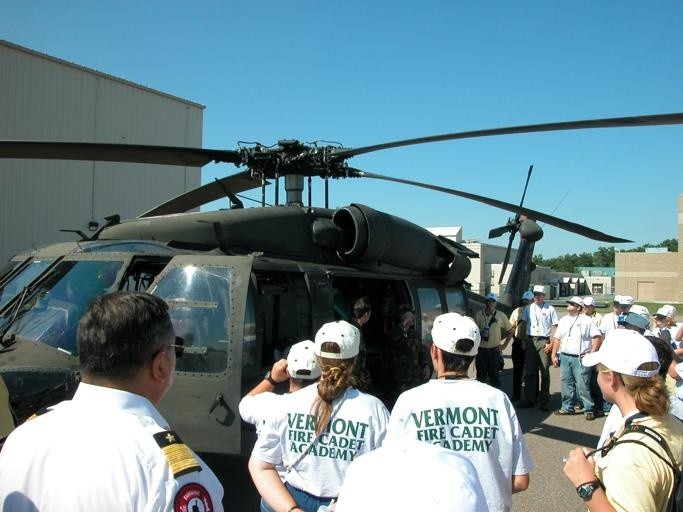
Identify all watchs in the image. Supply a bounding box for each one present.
[577,481,600,499]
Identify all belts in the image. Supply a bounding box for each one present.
[562,353,579,357]
[529,336,549,340]
[479,347,497,351]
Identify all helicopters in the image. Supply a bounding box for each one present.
[0,112,683,512]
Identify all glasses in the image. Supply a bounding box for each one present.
[166,336,184,358]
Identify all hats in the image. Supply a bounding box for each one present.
[533,285,545,295]
[485,293,497,302]
[315,320,360,359]
[583,297,595,307]
[427,312,481,356]
[566,296,584,309]
[620,296,634,305]
[613,295,622,304]
[286,340,322,380]
[522,291,534,300]
[624,305,650,324]
[582,329,661,377]
[617,312,650,330]
[663,305,676,316]
[653,308,670,318]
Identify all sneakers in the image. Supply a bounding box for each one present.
[595,409,603,418]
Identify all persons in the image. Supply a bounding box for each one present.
[552,295,603,420]
[248,321,391,511]
[509,291,535,402]
[476,293,514,386]
[383,311,531,511]
[616,312,648,337]
[599,294,622,341]
[520,284,558,410]
[563,329,682,511]
[238,338,321,483]
[352,298,371,388]
[2,290,224,511]
[394,307,433,396]
[584,297,605,417]
[651,307,674,341]
[617,296,633,315]
[595,335,682,463]
[628,305,649,322]
[661,305,682,330]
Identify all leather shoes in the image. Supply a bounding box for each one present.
[585,412,594,419]
[554,409,575,415]
[517,401,535,409]
[510,396,520,402]
[535,396,539,402]
[540,403,549,412]
